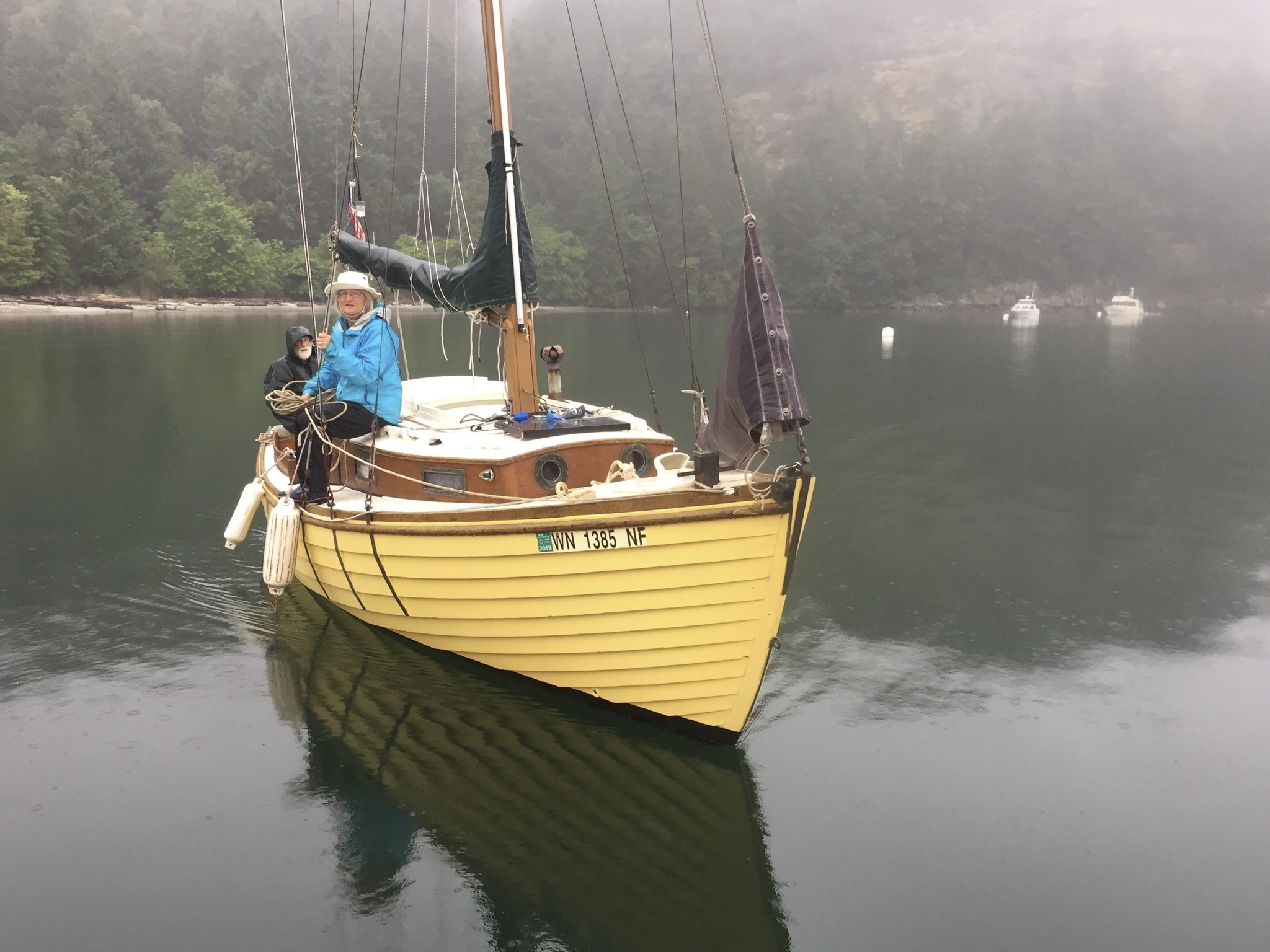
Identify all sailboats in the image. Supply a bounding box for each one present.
[256,2,825,750]
[260,566,793,952]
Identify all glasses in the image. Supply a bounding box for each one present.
[336,290,364,299]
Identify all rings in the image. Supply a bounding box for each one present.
[319,341,322,345]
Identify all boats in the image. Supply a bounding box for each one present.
[1105,287,1145,317]
[1011,295,1040,313]
[882,326,894,337]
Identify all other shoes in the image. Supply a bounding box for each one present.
[278,487,329,504]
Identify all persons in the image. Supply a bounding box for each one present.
[261,326,321,434]
[286,271,403,480]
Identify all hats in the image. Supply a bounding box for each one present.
[324,271,382,304]
